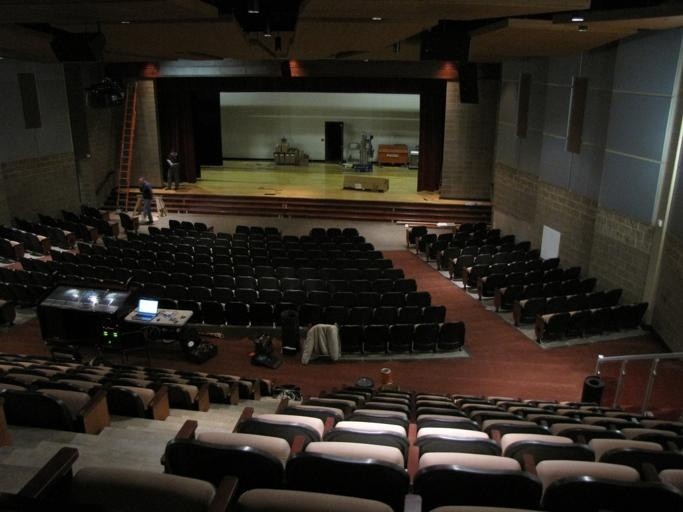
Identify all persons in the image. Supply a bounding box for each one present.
[138,177,154,225]
[164,148,181,190]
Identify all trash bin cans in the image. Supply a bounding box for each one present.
[582,375,605,402]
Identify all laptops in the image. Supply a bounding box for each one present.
[131,296,159,321]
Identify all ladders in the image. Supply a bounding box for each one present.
[116,81,138,212]
